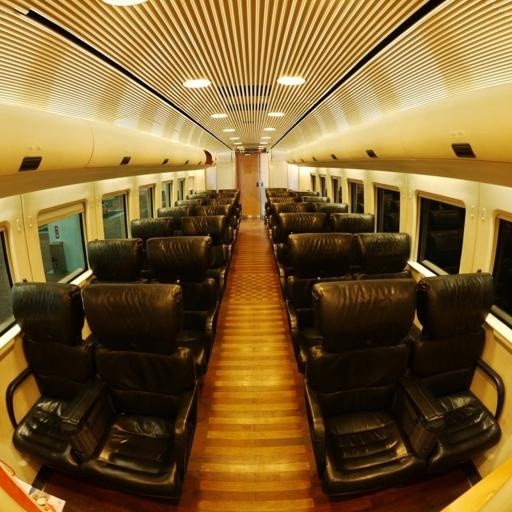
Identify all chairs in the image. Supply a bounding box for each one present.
[5,281,90,476]
[298,278,437,499]
[71,283,199,502]
[130,188,240,299]
[411,272,508,478]
[145,236,224,373]
[82,240,143,312]
[259,187,412,373]
